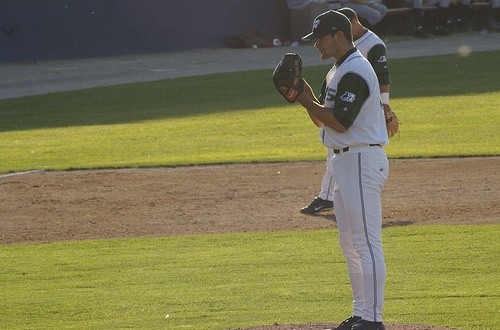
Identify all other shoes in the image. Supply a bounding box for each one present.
[345,319,385,330]
[300,197,334,214]
[332,316,361,330]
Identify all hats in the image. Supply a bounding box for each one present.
[336,8,356,20]
[302,10,351,40]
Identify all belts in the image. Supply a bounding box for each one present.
[334,144,381,154]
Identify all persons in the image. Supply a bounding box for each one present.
[274,10,389,330]
[286,0,500,42]
[300,8,400,214]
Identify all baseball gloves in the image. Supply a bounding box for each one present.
[274,51,307,104]
[382,104,399,136]
